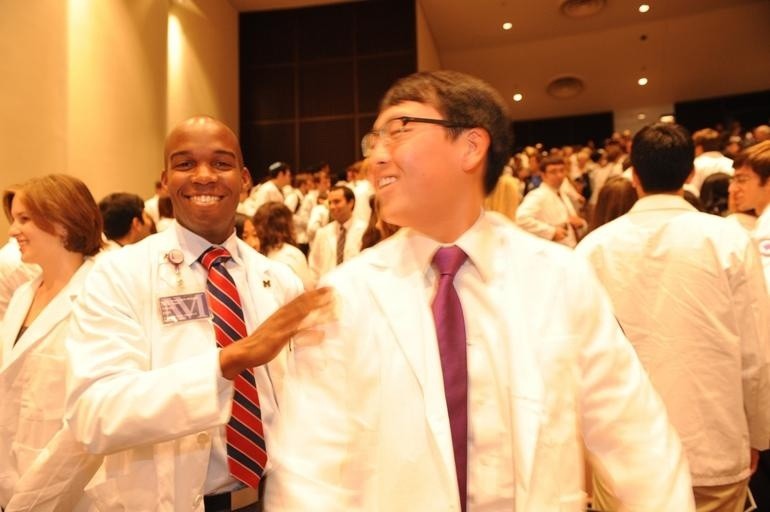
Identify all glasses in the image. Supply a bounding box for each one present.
[361,116,480,155]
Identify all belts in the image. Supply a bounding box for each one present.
[203,490,258,512]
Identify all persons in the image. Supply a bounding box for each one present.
[0,172,108,511]
[0,114,768,305]
[264,68,699,512]
[571,125,769,511]
[58,117,335,512]
[726,138,769,283]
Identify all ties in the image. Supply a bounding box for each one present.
[195,246,270,489]
[430,248,467,511]
[337,227,346,265]
